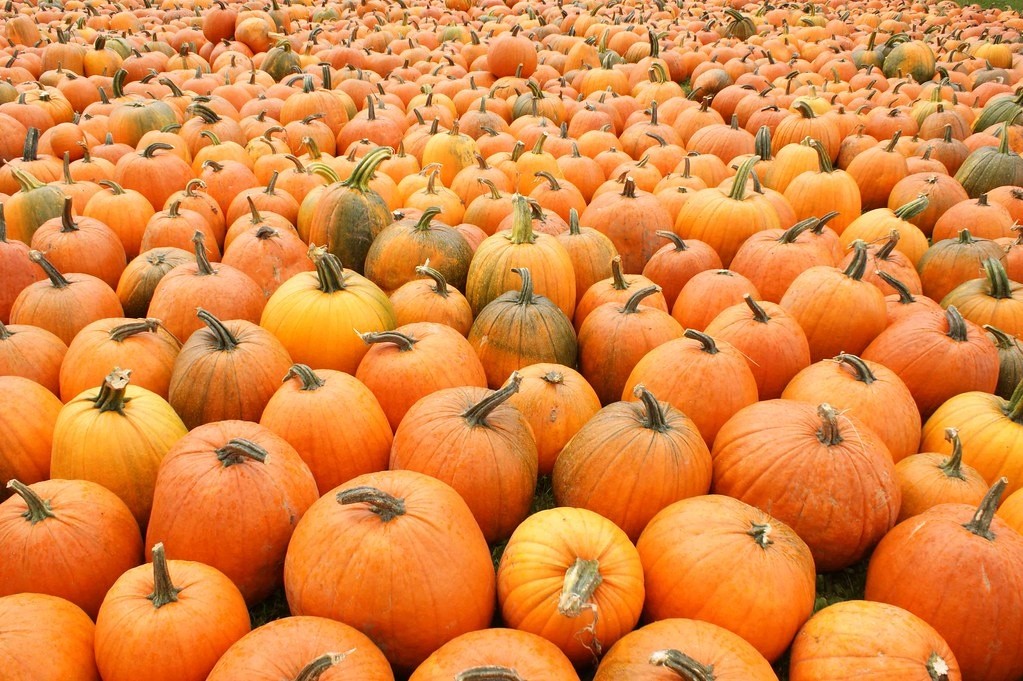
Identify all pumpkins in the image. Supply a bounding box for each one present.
[0,0,1023,681]
